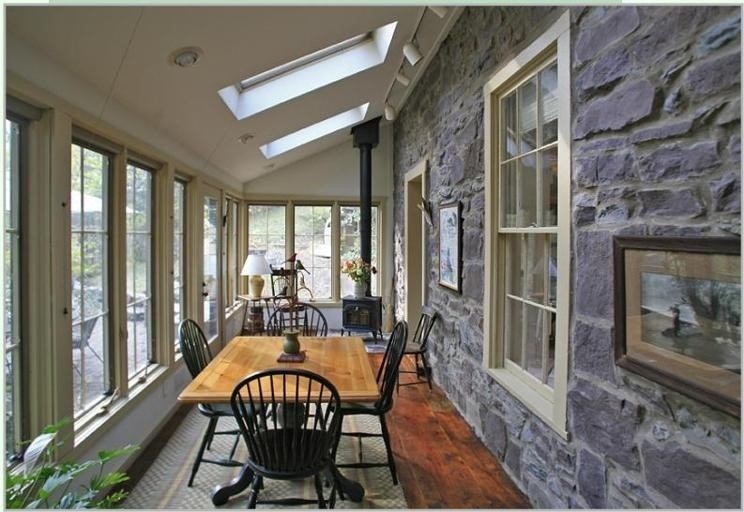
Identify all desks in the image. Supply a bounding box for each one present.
[341,295,383,345]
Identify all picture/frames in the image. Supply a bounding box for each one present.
[437,200,462,294]
[611,234,742,419]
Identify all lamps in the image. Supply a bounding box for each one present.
[240,256,273,302]
[386,102,395,121]
[429,5,449,18]
[168,46,205,69]
[404,42,424,66]
[204,255,216,283]
[397,73,410,86]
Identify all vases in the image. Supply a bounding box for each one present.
[353,281,366,299]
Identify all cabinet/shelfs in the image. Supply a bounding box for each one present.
[239,294,273,335]
[268,268,304,328]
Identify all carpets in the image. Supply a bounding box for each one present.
[104,401,407,508]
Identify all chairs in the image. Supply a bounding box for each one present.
[228,368,342,508]
[179,319,265,487]
[268,302,327,336]
[395,306,437,396]
[324,320,410,487]
[70,314,105,380]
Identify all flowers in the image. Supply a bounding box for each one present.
[340,257,379,281]
